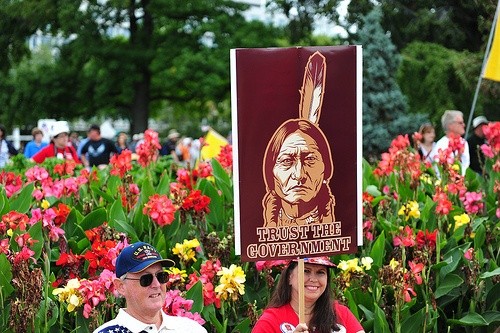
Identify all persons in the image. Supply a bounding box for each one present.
[91,242,208,333]
[249,256,364,333]
[0,122,212,171]
[407,109,490,183]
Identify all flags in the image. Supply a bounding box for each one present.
[481,12,500,82]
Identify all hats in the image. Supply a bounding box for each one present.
[48,125,70,143]
[286,255,337,267]
[472,116,490,128]
[115,242,176,278]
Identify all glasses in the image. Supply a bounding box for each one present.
[124,272,170,286]
